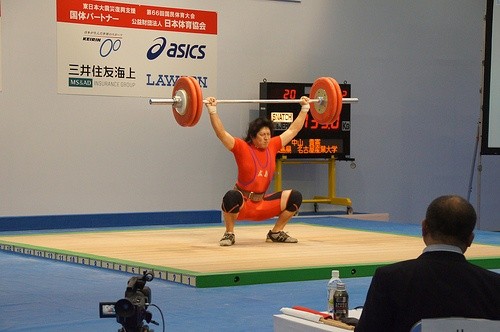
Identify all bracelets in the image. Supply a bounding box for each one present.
[208,106,217,114]
[301,105,310,112]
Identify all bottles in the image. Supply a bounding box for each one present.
[333,283,349,320]
[327,270,342,314]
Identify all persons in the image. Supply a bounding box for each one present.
[354,195,500,332]
[205,96,310,247]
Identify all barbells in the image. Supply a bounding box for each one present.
[149,76,360,128]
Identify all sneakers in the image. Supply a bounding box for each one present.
[266,229,298,244]
[220,232,236,247]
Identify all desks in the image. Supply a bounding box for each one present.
[272,309,363,332]
